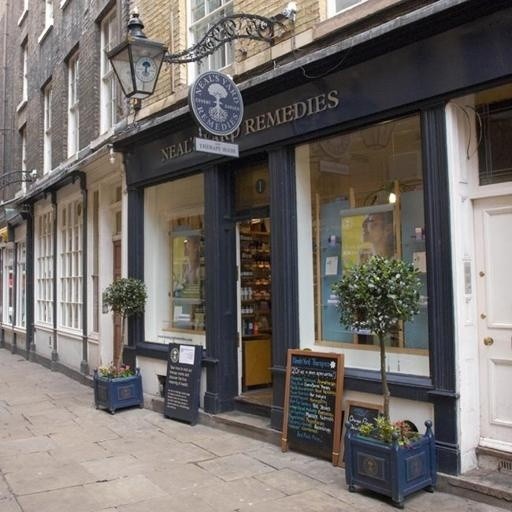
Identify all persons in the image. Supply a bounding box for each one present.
[357,213,395,266]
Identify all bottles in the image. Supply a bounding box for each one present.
[241,287,258,337]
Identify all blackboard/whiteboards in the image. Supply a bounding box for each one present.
[337,398,385,470]
[280,348,345,468]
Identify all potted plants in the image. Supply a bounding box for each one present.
[92,276,149,415]
[329,255,440,508]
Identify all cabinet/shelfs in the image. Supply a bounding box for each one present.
[240,257,257,319]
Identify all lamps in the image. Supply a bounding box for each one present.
[104,5,274,100]
